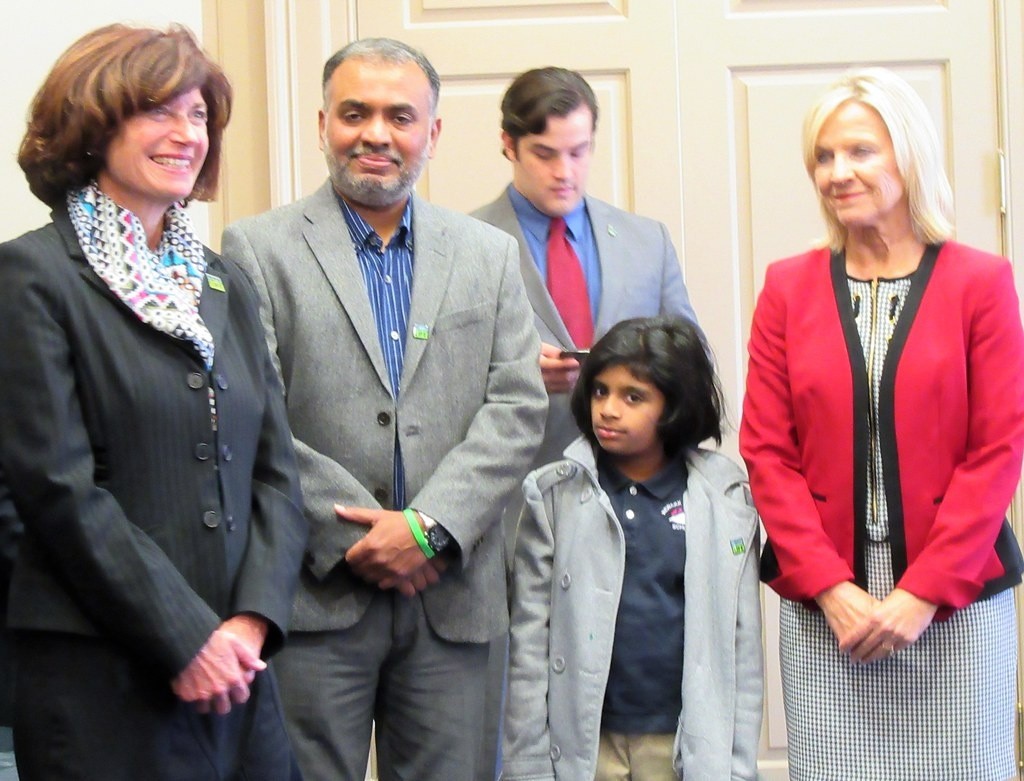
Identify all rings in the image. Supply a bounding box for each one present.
[883,644,893,650]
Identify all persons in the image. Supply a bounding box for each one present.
[502,315,765,781]
[470,66,698,572]
[221,35,550,781]
[0,24,312,781]
[739,68,1024,781]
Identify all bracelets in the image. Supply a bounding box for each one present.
[404,508,435,559]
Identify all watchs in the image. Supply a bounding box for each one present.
[420,511,450,551]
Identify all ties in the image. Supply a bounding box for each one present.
[546,217,593,351]
